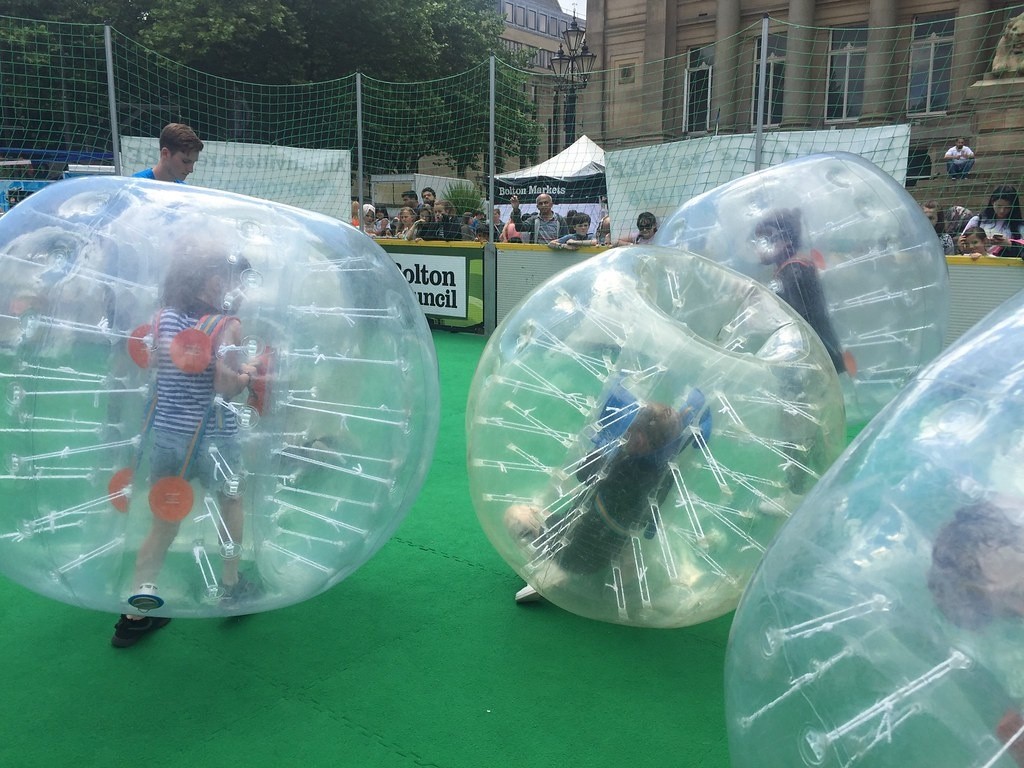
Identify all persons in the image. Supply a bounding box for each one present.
[956,227,1004,260]
[87,122,204,495]
[753,209,846,514]
[110,243,271,647]
[943,136,976,180]
[943,206,973,255]
[505,391,683,607]
[957,185,1024,259]
[915,200,955,257]
[928,502,1024,767]
[9,195,20,210]
[344,182,659,250]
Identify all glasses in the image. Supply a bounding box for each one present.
[638,222,654,231]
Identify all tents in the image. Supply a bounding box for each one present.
[485,135,608,240]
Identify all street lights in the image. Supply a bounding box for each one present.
[549,10,600,151]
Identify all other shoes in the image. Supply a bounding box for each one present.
[515,560,567,604]
[110,613,171,647]
[760,489,803,516]
[218,569,267,607]
